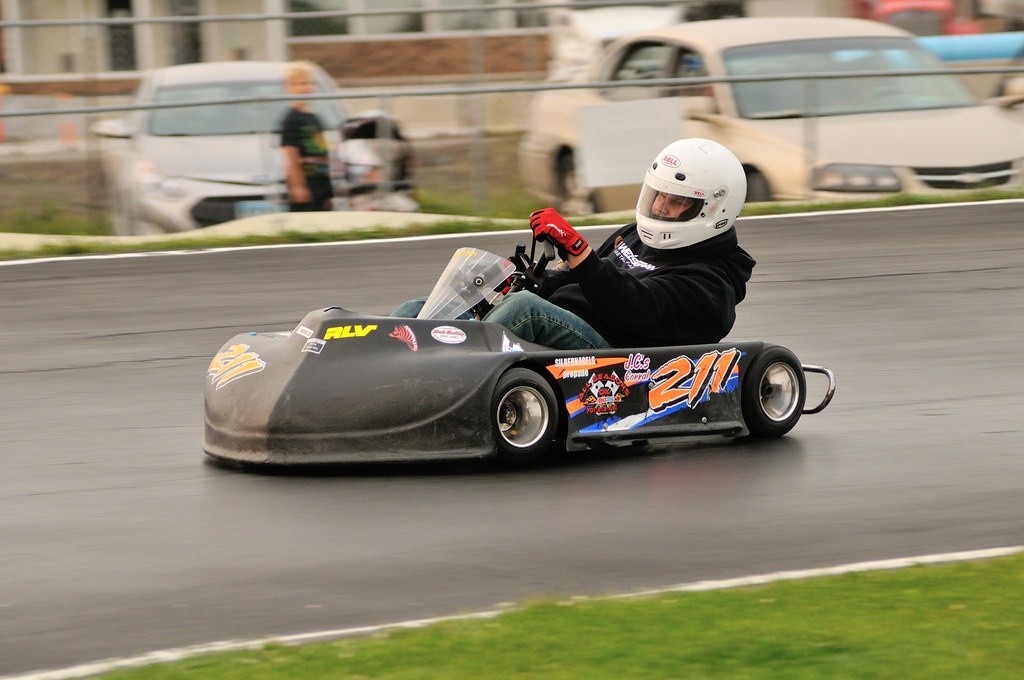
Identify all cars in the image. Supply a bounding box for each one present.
[82,60,420,238]
[855,2,990,39]
[514,14,1022,205]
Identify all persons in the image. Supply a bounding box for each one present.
[385,136,757,350]
[276,63,337,213]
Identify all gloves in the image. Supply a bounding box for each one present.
[529,207,588,258]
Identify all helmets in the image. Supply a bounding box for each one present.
[634,137,747,250]
